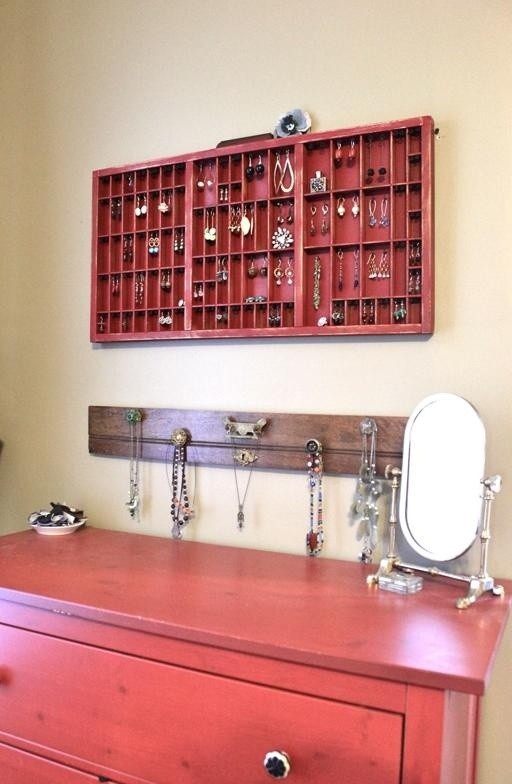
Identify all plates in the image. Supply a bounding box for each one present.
[32,518,86,536]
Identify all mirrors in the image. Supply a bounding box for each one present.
[397,393,486,561]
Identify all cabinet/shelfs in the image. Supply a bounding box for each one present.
[89,117,435,344]
[0,529,512,783]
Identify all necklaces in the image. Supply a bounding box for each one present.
[169,428,192,539]
[347,432,382,565]
[306,452,323,557]
[125,408,142,522]
[231,437,258,530]
